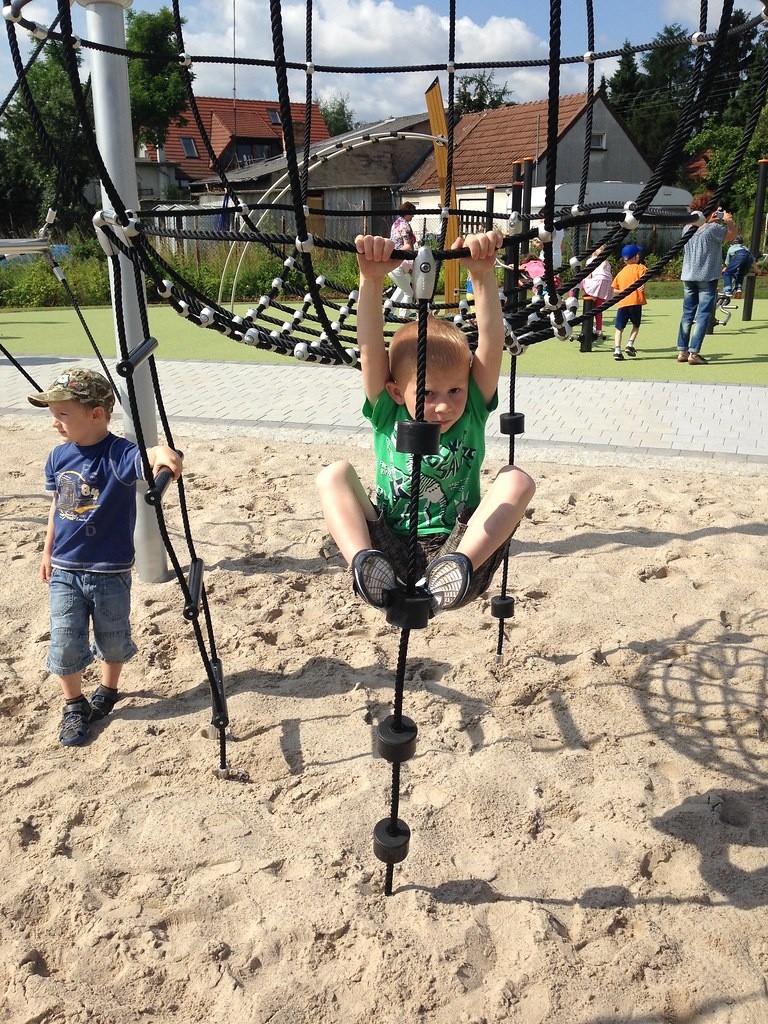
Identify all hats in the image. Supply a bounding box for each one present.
[622,243,644,259]
[731,237,740,244]
[27,367,115,412]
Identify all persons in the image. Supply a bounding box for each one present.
[466,266,477,326]
[381,201,416,327]
[27,368,184,746]
[530,209,566,278]
[717,235,761,300]
[676,191,737,365]
[610,244,648,360]
[317,230,537,615]
[569,247,614,339]
[507,252,559,328]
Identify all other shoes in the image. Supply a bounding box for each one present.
[625,346,637,356]
[613,352,624,359]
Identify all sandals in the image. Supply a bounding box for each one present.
[689,353,709,364]
[678,350,688,362]
[87,684,119,726]
[60,696,89,744]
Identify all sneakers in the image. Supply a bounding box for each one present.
[414,550,473,616]
[734,286,742,298]
[717,287,732,296]
[351,547,408,612]
